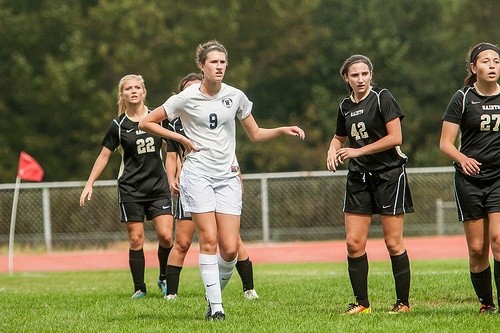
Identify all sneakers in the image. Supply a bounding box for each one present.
[389,302,410,315]
[164,293,179,300]
[158,279,167,294]
[131,290,145,298]
[244,289,258,299]
[205,304,212,318]
[479,304,495,313]
[344,302,371,314]
[210,311,225,320]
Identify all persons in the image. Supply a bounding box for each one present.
[439,42,500,317]
[138,41,305,321]
[79,71,258,301]
[327,55,414,316]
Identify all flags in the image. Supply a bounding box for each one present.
[17,151,45,183]
